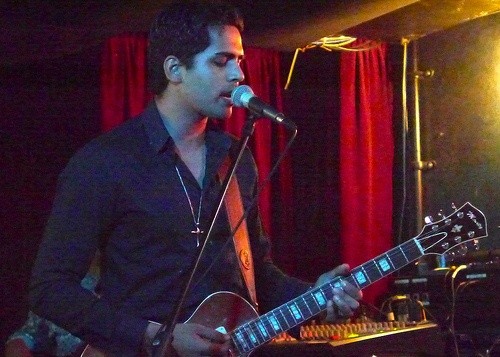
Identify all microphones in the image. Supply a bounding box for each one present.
[230,84,298,131]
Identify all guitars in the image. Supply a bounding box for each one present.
[81,202,488,357]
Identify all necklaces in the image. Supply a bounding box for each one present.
[163,106,211,247]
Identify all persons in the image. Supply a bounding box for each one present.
[20,1,364,356]
[3,229,103,357]
